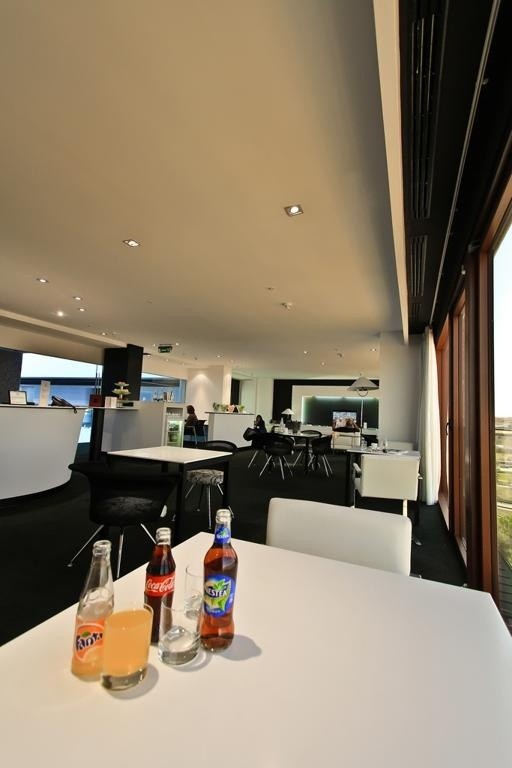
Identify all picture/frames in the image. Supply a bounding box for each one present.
[8,389,27,405]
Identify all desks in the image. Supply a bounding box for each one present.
[107,445,234,548]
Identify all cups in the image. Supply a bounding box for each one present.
[185,562,208,619]
[157,589,205,668]
[101,602,155,692]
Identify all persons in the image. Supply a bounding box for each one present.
[255,415,267,433]
[183,406,200,428]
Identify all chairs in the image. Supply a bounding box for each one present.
[65,457,176,580]
[170,440,239,531]
[265,496,413,575]
[184,420,207,446]
[331,430,361,449]
[243,422,336,481]
[346,435,424,548]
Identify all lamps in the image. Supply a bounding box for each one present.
[346,375,379,428]
[281,408,295,422]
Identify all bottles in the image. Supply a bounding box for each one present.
[198,509,238,654]
[70,538,116,682]
[143,527,177,645]
